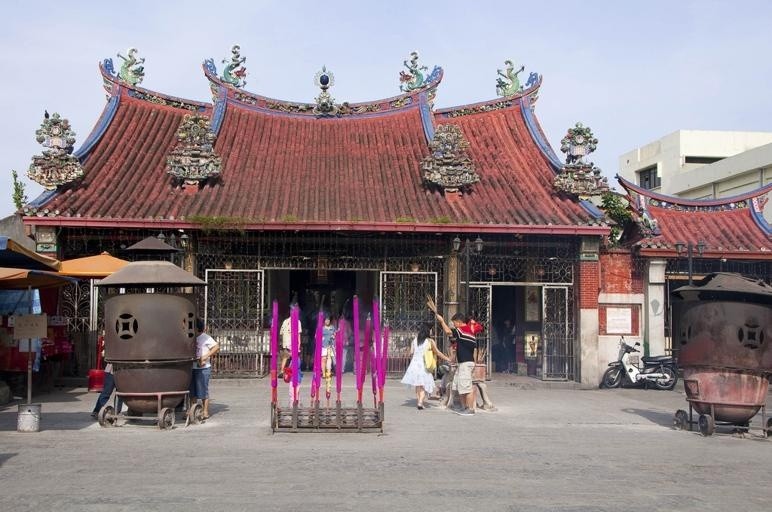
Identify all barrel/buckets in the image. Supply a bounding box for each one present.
[17,404,41,432]
[87,369,104,392]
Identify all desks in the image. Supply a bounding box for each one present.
[2,340,79,395]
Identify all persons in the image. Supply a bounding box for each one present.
[184,319,219,419]
[401,315,516,415]
[90,346,123,418]
[314,315,336,378]
[336,314,352,373]
[280,305,302,377]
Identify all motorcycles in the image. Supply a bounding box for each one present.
[599,336,679,392]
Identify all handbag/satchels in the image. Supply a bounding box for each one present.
[423,339,438,374]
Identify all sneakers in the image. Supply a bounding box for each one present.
[200,414,210,422]
[454,407,476,417]
[278,371,284,378]
[90,411,99,421]
[416,400,426,410]
